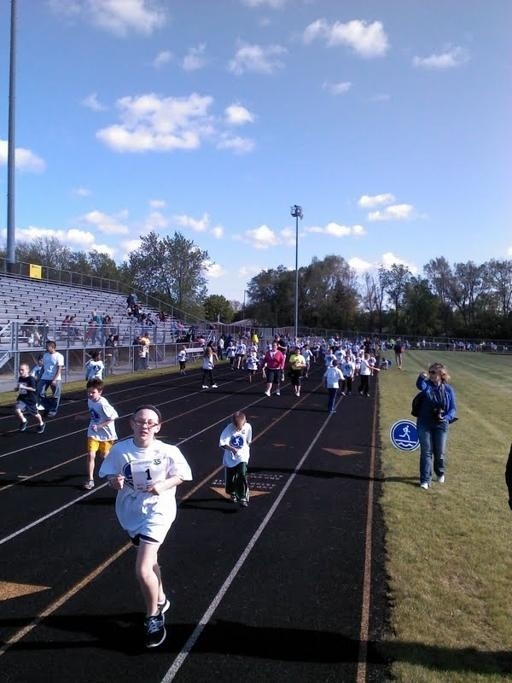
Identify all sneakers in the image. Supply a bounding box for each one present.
[144,595,171,648]
[39,423,46,433]
[84,480,94,490]
[231,493,248,511]
[21,419,28,432]
[420,474,444,489]
[202,384,218,389]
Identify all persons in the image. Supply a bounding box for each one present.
[450,340,511,353]
[125,291,168,325]
[174,318,410,413]
[97,402,194,648]
[12,341,64,434]
[84,377,120,490]
[417,337,441,349]
[13,314,115,345]
[218,410,253,507]
[132,331,151,370]
[504,442,511,512]
[85,333,119,381]
[414,361,457,490]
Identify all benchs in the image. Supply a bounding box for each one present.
[0,276,219,343]
[185,347,218,362]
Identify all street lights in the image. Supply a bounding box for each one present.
[290,203,304,341]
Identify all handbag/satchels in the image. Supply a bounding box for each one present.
[411,392,424,417]
